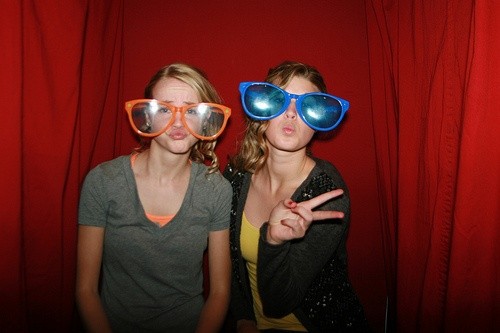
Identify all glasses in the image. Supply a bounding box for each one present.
[124,97,232,140]
[238,81,349,130]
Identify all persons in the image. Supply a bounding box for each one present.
[76,64,231,333]
[223,60,371,333]
